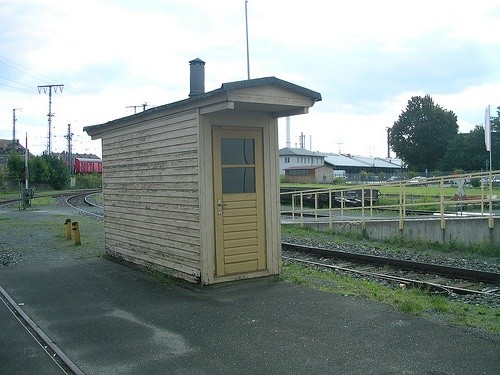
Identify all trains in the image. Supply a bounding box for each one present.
[280,185,380,208]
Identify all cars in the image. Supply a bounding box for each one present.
[386,175,500,188]
